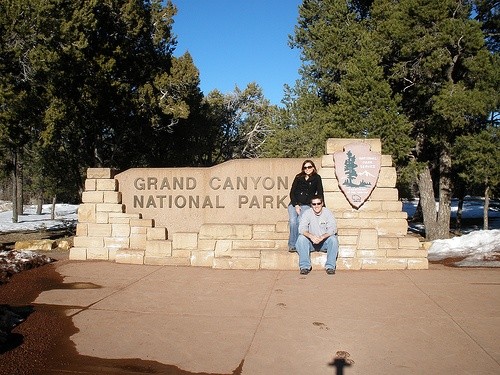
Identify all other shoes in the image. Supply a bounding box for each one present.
[326,269,335,274]
[300,269,308,274]
[289,248,297,252]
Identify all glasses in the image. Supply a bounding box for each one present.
[303,166,314,170]
[312,201,322,205]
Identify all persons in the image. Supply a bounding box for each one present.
[296,197,339,274]
[287,160,324,252]
